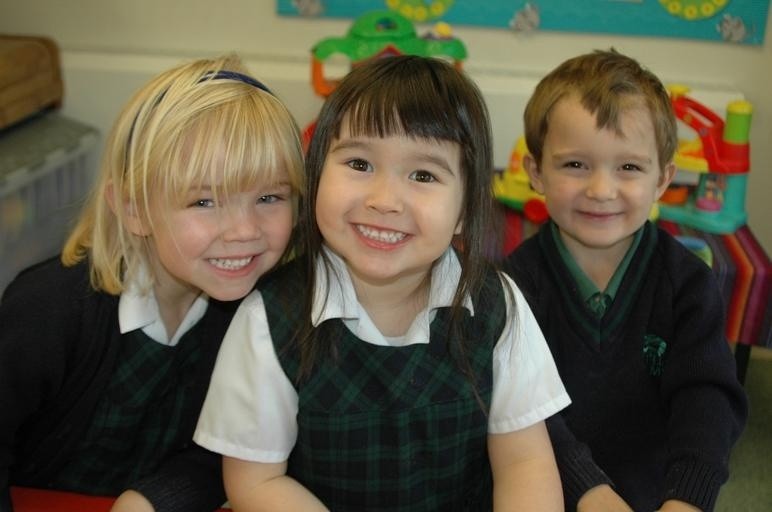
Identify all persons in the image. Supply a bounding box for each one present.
[192,53,573,512]
[1,51,310,512]
[500,46,749,512]
